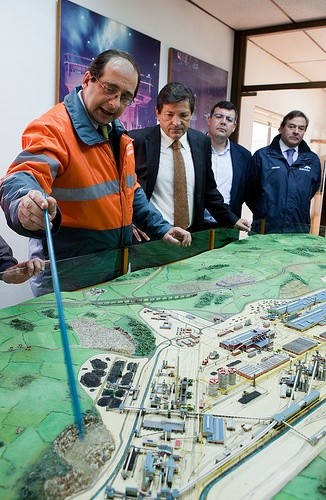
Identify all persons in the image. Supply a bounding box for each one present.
[0,49,193,297]
[0,233,51,285]
[247,109,321,235]
[199,99,252,252]
[125,81,252,274]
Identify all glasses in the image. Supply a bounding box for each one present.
[93,75,135,106]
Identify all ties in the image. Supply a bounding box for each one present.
[102,125,109,140]
[285,148,296,166]
[173,140,190,230]
